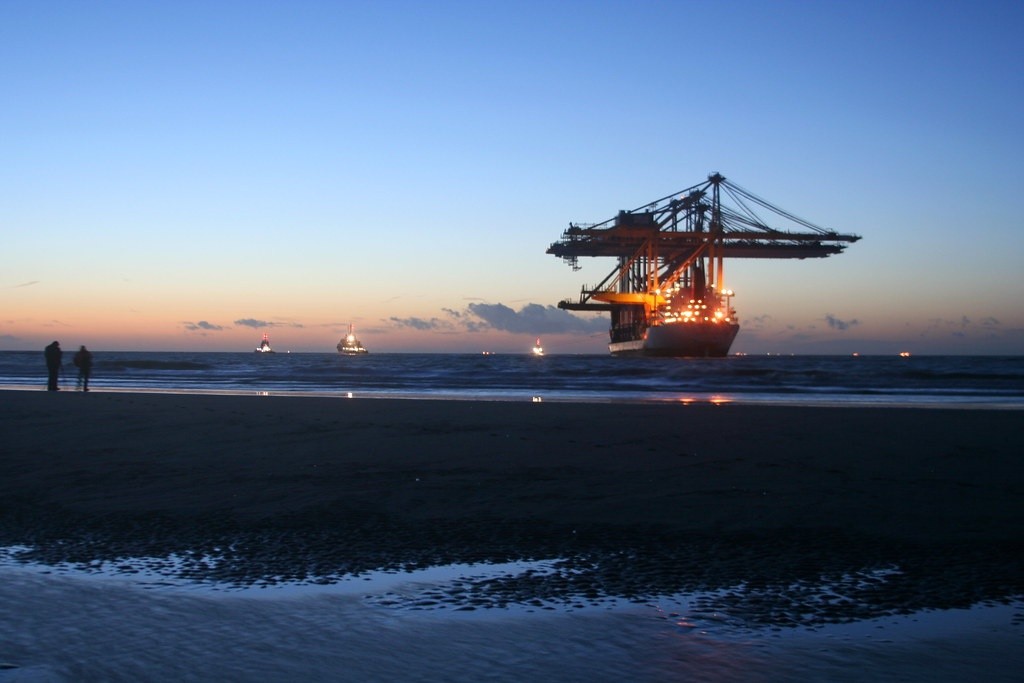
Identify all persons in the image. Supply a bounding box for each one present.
[74,345,94,390]
[44,341,63,391]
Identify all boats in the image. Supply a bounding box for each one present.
[546,171,863,357]
[337,323,368,353]
[255,334,272,353]
